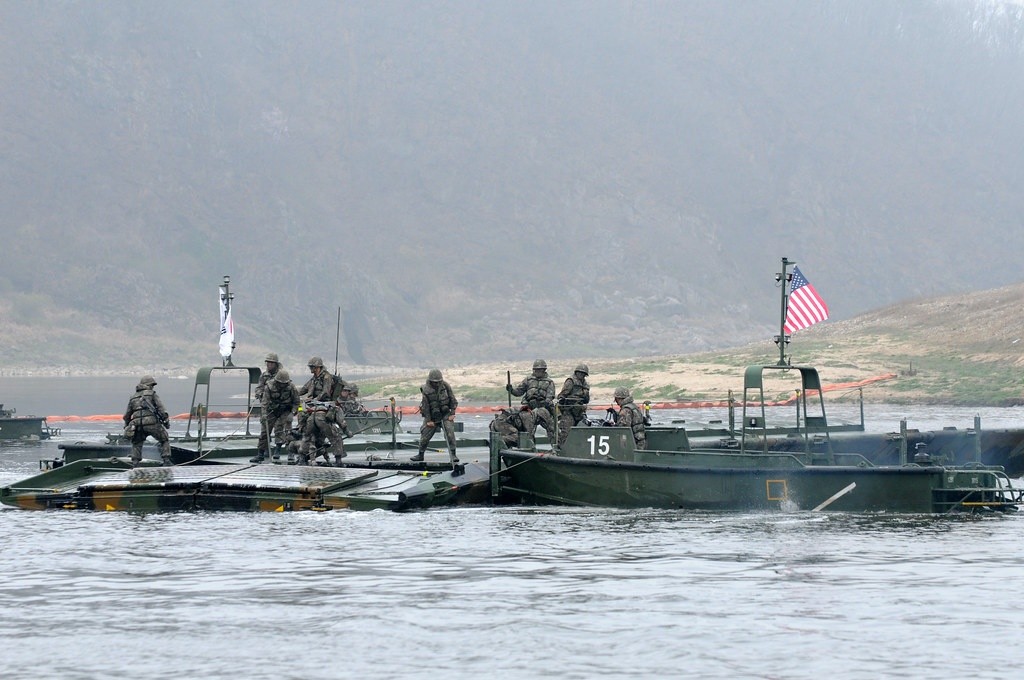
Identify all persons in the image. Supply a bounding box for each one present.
[123,375,175,466]
[488,406,533,448]
[298,357,333,467]
[606,386,646,451]
[409,369,460,463]
[250,371,301,464]
[336,383,367,414]
[557,364,591,451]
[506,360,557,447]
[254,353,283,458]
[285,439,318,466]
[298,401,353,468]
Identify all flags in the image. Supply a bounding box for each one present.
[219,287,235,357]
[783,265,829,335]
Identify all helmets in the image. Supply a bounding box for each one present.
[537,407,550,424]
[614,387,630,399]
[347,382,359,394]
[342,380,350,390]
[141,375,157,386]
[307,357,324,367]
[574,364,589,376]
[532,359,547,370]
[428,368,443,382]
[265,352,279,362]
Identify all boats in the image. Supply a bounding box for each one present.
[0,403,62,443]
[487,258,1024,522]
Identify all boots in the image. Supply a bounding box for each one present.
[449,449,459,462]
[273,444,282,459]
[163,458,174,466]
[249,450,264,463]
[335,455,344,467]
[410,452,424,461]
[130,464,138,469]
[298,454,306,466]
[287,450,295,460]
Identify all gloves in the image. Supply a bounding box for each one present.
[506,384,513,392]
[559,398,565,404]
[606,407,616,414]
[603,422,612,427]
[164,422,170,428]
[544,402,549,409]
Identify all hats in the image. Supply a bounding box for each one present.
[274,370,291,383]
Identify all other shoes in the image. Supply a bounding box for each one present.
[263,449,269,458]
